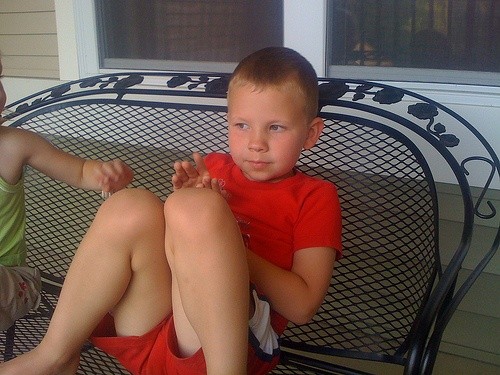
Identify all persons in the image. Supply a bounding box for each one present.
[0,54,136,333]
[1,45,345,375]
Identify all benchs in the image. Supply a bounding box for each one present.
[0,69,500,375]
